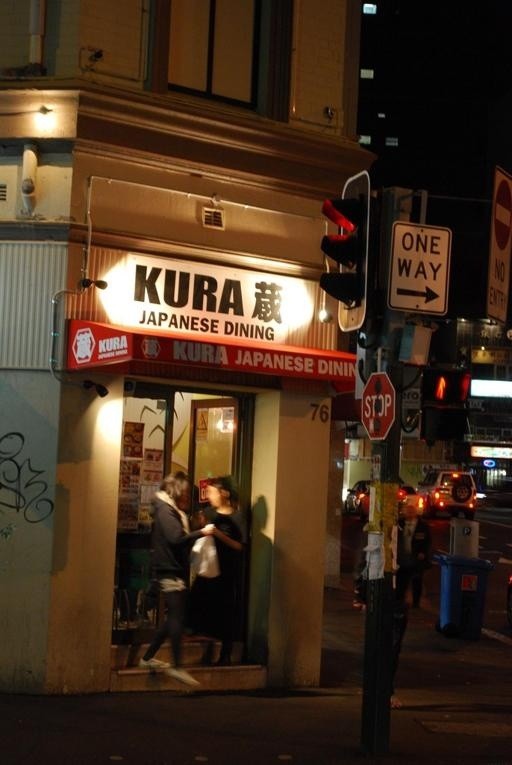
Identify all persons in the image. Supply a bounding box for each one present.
[140,473,216,687]
[396,504,431,609]
[184,476,250,667]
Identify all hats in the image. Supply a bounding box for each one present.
[217,475,240,501]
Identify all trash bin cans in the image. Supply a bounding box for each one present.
[433,550,493,640]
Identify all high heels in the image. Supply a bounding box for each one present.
[199,643,232,667]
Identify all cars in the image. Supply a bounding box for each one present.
[345,470,477,523]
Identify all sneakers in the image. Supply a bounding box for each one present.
[139,657,198,687]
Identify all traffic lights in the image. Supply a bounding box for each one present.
[319,169,376,333]
[421,368,472,440]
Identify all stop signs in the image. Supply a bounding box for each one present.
[362,372,397,441]
[486,165,512,325]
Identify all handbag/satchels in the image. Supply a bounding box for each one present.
[144,535,180,570]
[191,535,220,578]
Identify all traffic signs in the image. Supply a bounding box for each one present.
[386,221,453,316]
